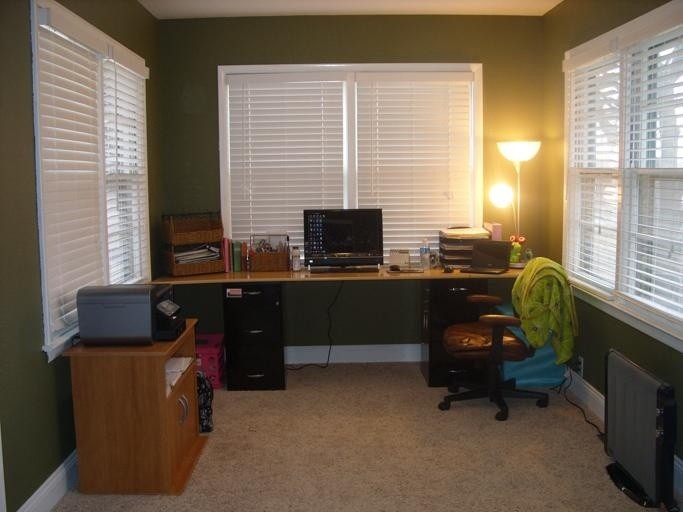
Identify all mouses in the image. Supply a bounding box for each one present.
[444,266,454,273]
[390,265,400,271]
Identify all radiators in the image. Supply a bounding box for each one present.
[603,347,673,506]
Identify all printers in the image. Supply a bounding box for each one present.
[77,283,186,345]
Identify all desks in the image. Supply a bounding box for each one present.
[155,263,557,393]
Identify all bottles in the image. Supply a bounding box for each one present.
[525,249,533,263]
[292,247,300,271]
[420,238,430,270]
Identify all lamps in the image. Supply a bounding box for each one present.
[490,138,543,256]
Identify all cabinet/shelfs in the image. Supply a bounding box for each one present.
[64,317,208,497]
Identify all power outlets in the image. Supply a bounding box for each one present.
[577,356,584,377]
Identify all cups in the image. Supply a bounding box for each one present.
[389,250,410,266]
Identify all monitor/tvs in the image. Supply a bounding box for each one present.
[304,208,383,266]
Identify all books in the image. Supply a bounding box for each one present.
[174,238,250,274]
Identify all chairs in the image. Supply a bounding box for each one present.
[442,255,574,425]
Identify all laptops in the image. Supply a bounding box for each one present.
[460,240,511,274]
